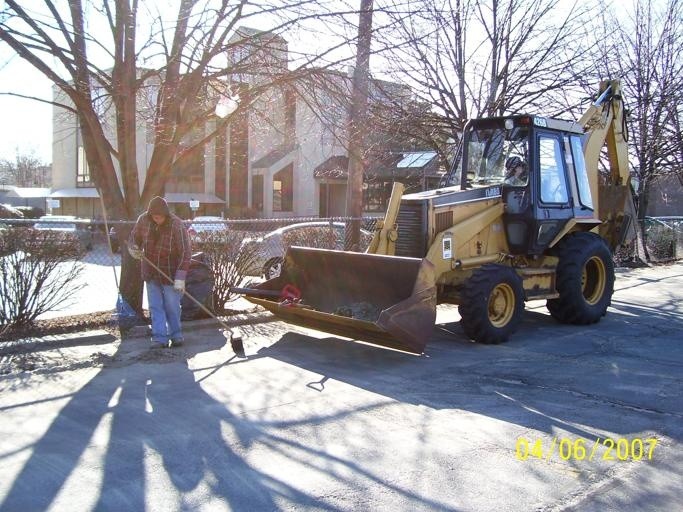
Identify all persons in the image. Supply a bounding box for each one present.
[127,196,191,349]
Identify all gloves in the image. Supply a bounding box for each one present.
[173,279,186,293]
[128,244,144,260]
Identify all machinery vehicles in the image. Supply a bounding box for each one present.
[236,76,641,356]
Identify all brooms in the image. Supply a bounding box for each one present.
[123,239,243,349]
[98,187,137,317]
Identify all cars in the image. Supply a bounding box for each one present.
[183,216,232,252]
[236,221,375,283]
[0,202,46,256]
[22,215,96,260]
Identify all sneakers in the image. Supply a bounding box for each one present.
[149,336,184,349]
